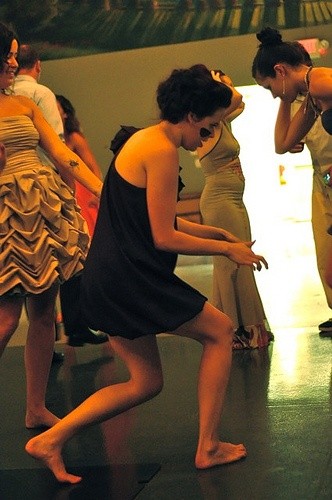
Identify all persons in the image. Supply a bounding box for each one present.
[191,68,275,349]
[24,64,269,484]
[251,26,332,332]
[0,23,104,429]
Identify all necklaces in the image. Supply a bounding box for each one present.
[304,66,323,121]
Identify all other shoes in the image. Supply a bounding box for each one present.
[319,318,332,331]
[52,351,64,361]
[67,328,109,346]
